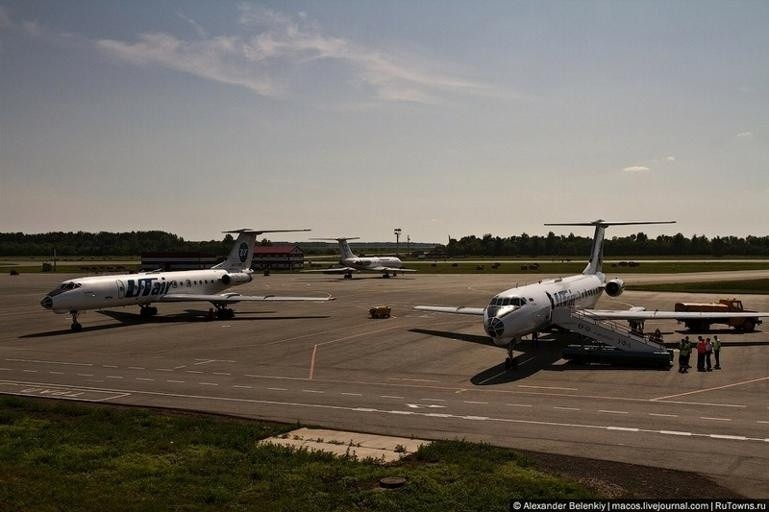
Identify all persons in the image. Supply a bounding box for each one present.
[677,335,723,374]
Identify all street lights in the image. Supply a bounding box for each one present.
[394,228,402,258]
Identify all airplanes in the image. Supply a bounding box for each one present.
[300,237,418,279]
[40,229,336,330]
[413,220,769,368]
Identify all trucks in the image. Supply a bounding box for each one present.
[676,299,762,332]
